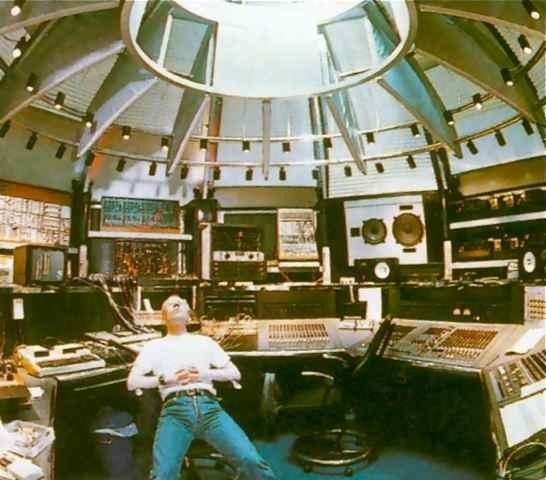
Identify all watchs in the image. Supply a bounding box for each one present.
[159,374,167,385]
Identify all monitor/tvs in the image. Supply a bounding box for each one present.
[13,244,68,286]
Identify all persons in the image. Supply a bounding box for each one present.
[126,295,276,479]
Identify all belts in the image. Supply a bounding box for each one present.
[163,387,219,404]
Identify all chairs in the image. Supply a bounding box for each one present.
[287,315,394,474]
[138,383,246,480]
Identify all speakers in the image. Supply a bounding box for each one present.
[353,257,400,282]
[517,245,545,283]
[342,194,428,267]
